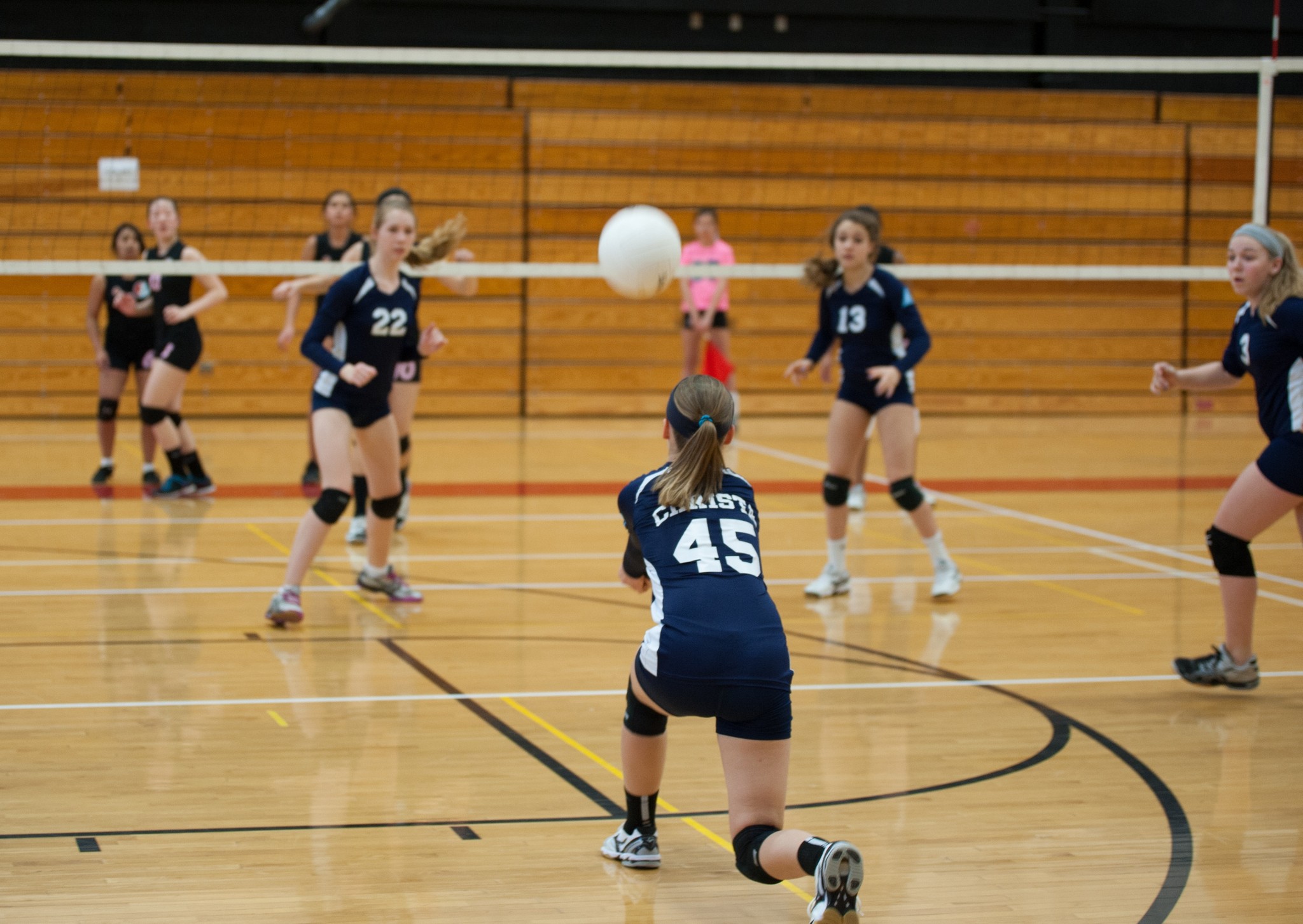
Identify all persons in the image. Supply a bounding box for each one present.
[601,381,863,923]
[680,208,741,427]
[780,207,959,600]
[1150,224,1303,688]
[87,187,466,628]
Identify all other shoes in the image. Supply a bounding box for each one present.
[193,477,213,495]
[154,475,197,497]
[916,481,935,505]
[848,482,866,511]
[93,465,112,483]
[345,514,368,545]
[143,471,161,484]
[396,488,407,530]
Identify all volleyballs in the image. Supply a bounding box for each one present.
[598,204,682,299]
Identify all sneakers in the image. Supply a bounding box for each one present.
[806,565,851,599]
[601,820,663,869]
[930,564,962,598]
[1174,643,1259,688]
[357,563,422,602]
[806,841,865,923]
[264,589,305,625]
